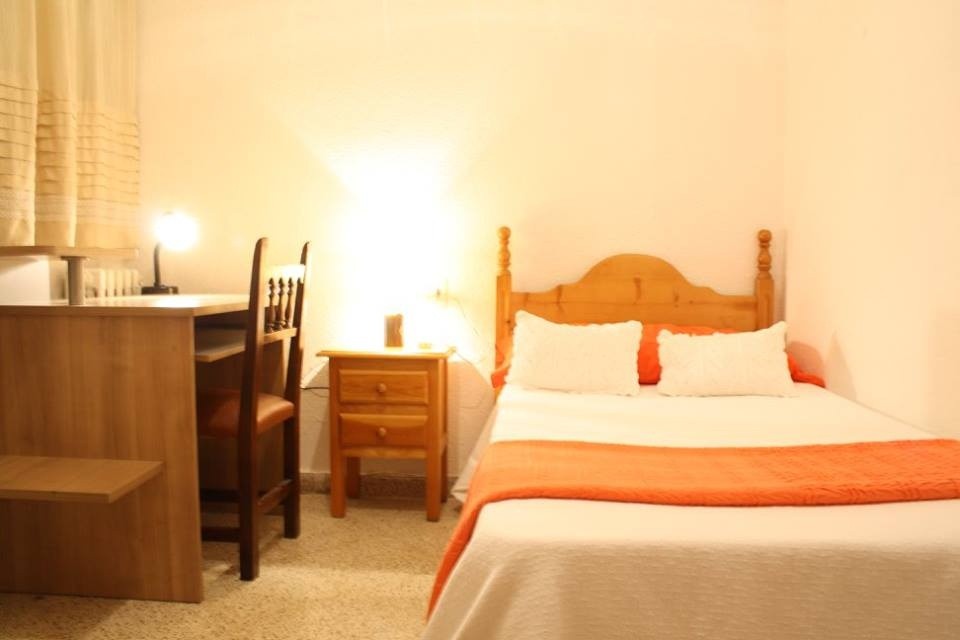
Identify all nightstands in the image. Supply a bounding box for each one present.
[315,346,457,522]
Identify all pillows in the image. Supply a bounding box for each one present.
[654,321,798,398]
[491,324,827,389]
[503,309,643,395]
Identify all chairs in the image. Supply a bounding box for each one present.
[196,237,310,582]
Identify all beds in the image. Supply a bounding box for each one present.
[423,226,960,640]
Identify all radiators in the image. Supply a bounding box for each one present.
[83,267,138,295]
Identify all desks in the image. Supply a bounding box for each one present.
[0,295,290,604]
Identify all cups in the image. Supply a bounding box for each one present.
[382,311,404,351]
